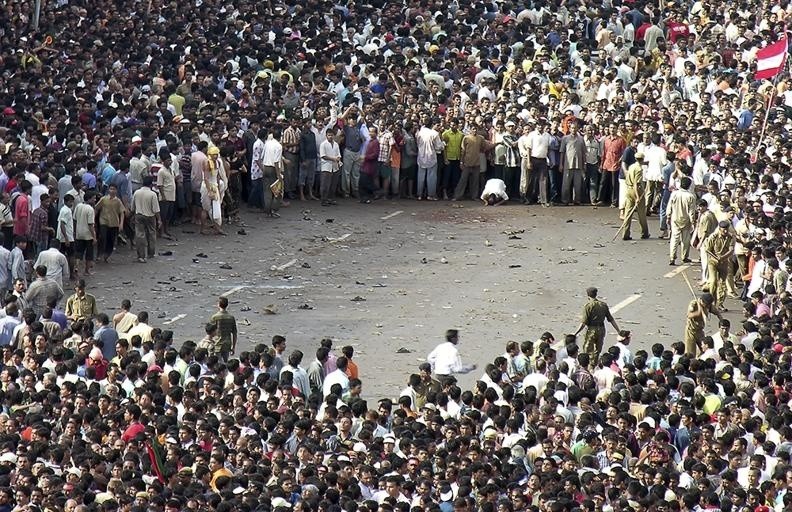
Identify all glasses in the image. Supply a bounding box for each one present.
[0,364,792,512]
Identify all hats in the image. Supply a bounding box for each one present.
[702,293,714,304]
[76,280,85,289]
[0,0,792,243]
[587,287,598,297]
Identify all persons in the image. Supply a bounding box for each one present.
[0,2,792,511]
